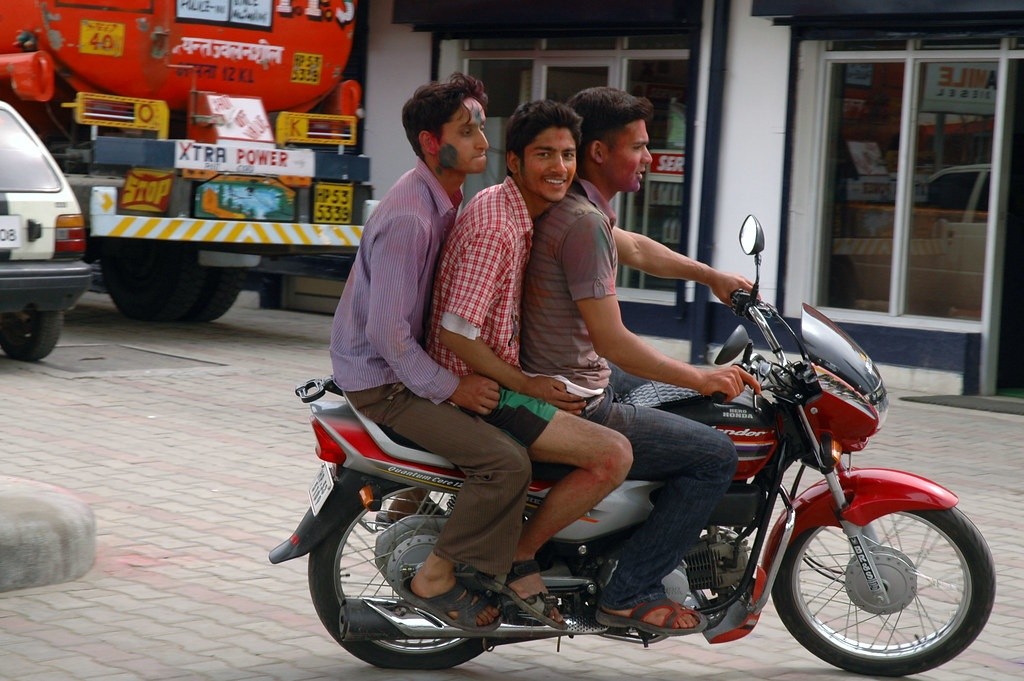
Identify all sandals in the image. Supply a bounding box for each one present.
[475,561,568,633]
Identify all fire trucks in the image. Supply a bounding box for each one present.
[0,1,373,324]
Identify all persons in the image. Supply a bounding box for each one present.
[327,72,532,633]
[520,87,761,636]
[424,99,634,630]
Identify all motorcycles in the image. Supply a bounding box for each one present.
[266,357,995,678]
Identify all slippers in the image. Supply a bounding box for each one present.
[399,571,502,636]
[597,591,710,637]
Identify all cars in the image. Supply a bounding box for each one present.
[854,162,988,319]
[1,100,91,364]
